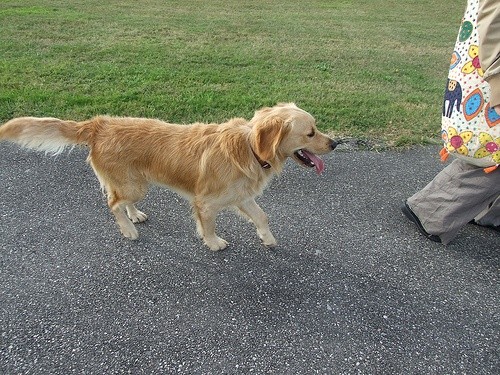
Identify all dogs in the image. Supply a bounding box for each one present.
[1,100,337,251]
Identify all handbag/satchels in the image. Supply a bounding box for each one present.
[401,200,441,242]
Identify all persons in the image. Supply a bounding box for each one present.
[399,1,500,243]
